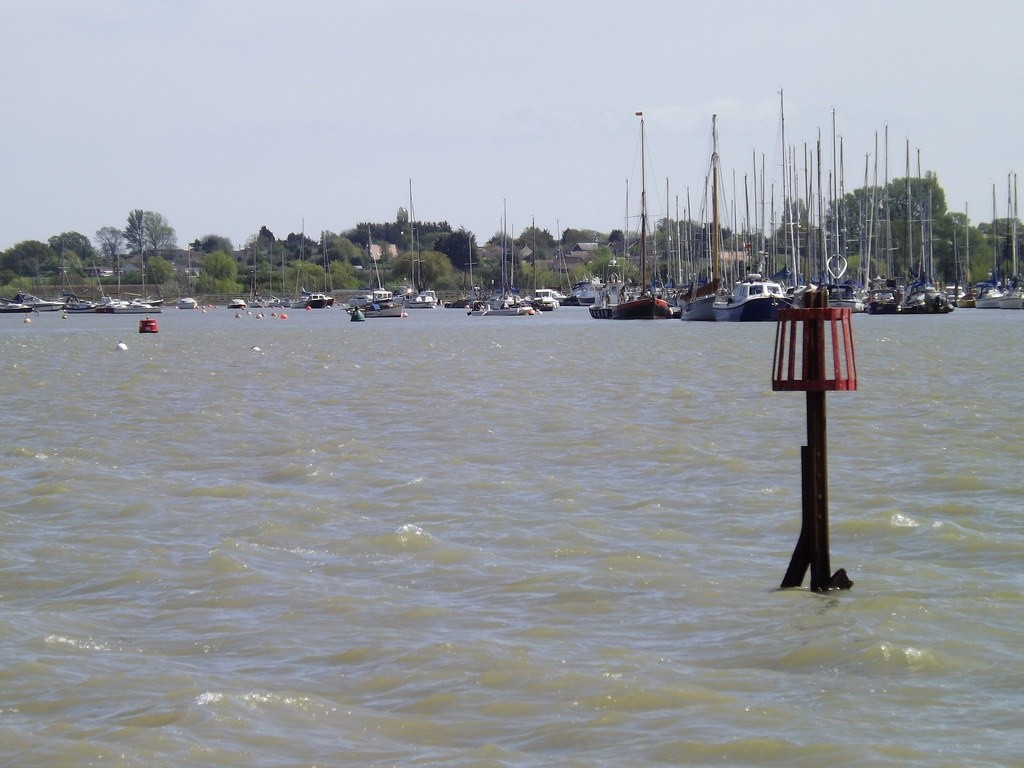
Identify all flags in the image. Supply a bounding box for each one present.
[636,112,642,115]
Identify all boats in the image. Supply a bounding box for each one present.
[345,298,403,317]
[228,299,247,309]
[10,287,64,312]
[0,295,32,313]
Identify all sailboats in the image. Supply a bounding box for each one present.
[444,199,595,316]
[348,177,439,308]
[177,245,197,310]
[587,87,1024,323]
[34,224,164,314]
[248,218,336,309]
